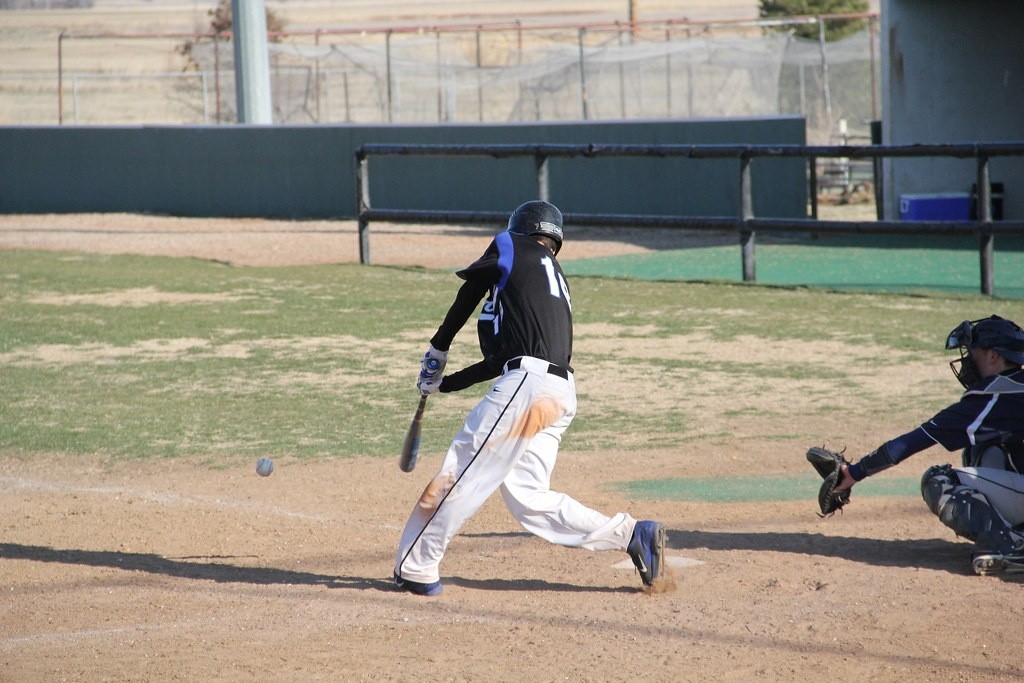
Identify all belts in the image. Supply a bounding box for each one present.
[500,358,569,380]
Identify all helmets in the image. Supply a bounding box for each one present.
[506,200,564,245]
[967,318,1024,366]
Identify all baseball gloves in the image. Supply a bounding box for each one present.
[806,448,851,517]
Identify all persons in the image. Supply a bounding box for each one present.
[825,315,1024,577]
[394,202,670,597]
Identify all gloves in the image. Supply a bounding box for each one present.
[415,374,447,396]
[417,344,450,376]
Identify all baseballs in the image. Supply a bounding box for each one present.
[399,358,439,473]
[255,458,274,477]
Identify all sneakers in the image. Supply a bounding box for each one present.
[625,519,669,587]
[971,539,1024,576]
[391,568,444,597]
